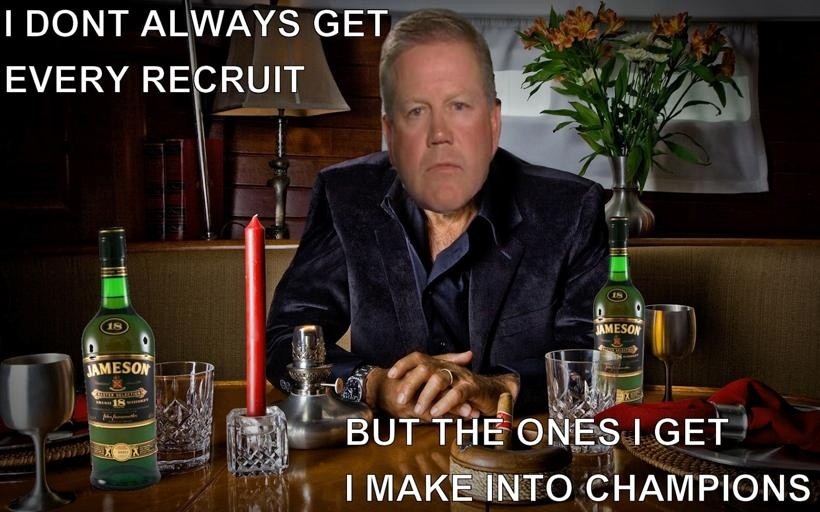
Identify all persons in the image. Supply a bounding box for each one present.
[494,392,513,450]
[265,8,610,426]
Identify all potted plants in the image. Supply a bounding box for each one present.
[598,153,657,235]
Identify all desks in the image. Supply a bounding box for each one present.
[10,367,813,508]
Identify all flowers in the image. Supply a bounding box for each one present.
[522,2,741,194]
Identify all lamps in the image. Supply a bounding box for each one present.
[212,4,357,239]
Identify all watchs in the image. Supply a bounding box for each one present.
[340,363,379,402]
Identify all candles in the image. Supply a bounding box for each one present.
[245,212,266,419]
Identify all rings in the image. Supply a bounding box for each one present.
[439,368,453,385]
